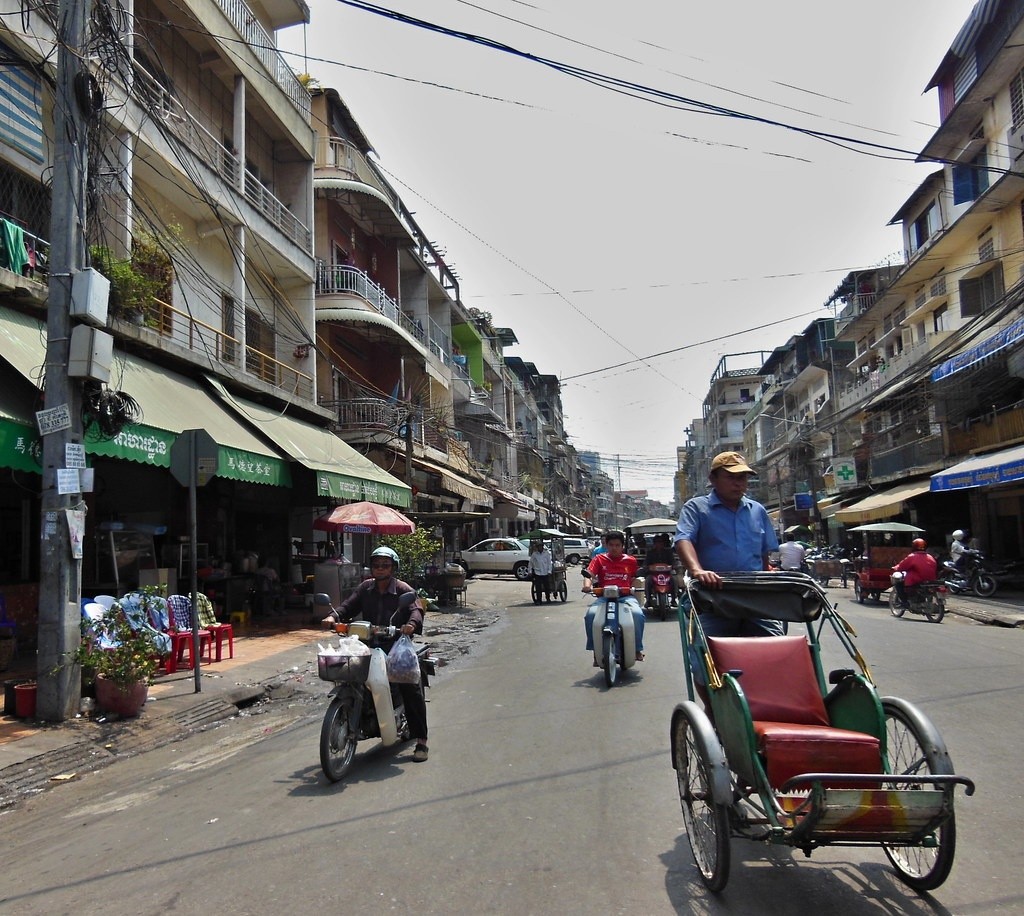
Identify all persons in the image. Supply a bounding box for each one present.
[950,529,982,581]
[528,540,552,604]
[674,451,785,724]
[638,532,678,608]
[892,538,937,605]
[582,531,645,667]
[778,534,806,571]
[322,547,429,762]
[591,534,609,559]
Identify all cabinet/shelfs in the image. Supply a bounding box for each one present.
[96,530,157,586]
[162,543,208,580]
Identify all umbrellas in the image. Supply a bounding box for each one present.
[313,501,415,566]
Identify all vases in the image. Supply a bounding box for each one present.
[4,679,37,717]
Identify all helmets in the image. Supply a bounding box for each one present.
[369,547,400,569]
[952,530,964,541]
[913,538,927,550]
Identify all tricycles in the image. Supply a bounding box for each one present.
[667,571,978,889]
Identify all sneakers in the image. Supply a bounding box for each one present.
[413,744,429,762]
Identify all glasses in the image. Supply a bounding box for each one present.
[373,563,392,569]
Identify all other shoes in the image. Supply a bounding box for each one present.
[386,634,421,684]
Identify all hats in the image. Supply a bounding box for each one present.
[710,452,758,475]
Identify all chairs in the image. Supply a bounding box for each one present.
[0,592,19,659]
[694,635,887,793]
[81,591,233,673]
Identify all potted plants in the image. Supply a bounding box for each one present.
[51,583,168,713]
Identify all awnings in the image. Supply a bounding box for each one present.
[860,316,1024,411]
[387,448,494,508]
[930,445,1024,492]
[484,484,521,504]
[0,394,44,475]
[212,387,412,508]
[835,479,932,522]
[0,306,296,490]
[766,505,796,531]
[818,493,846,512]
[821,496,860,519]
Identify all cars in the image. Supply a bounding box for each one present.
[455,538,536,580]
[535,539,595,564]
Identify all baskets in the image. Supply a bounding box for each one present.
[317,654,372,683]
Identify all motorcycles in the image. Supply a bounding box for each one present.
[318,589,446,783]
[844,521,927,604]
[622,517,689,620]
[886,561,947,625]
[941,546,996,599]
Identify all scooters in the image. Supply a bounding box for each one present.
[577,568,647,687]
